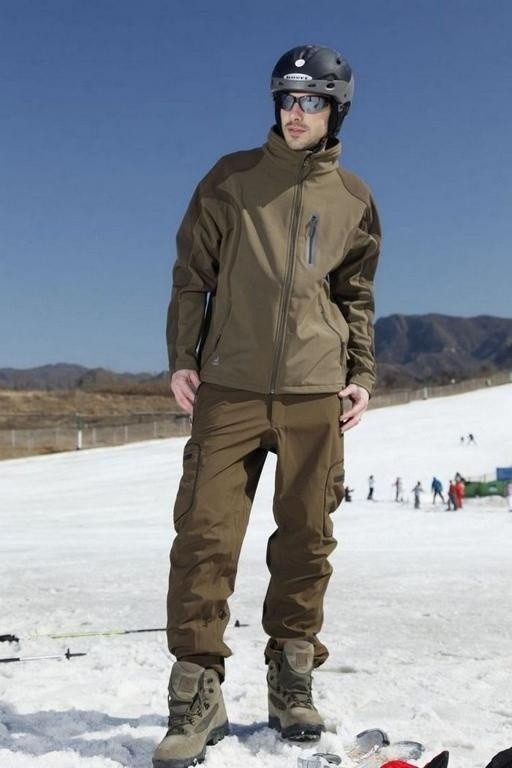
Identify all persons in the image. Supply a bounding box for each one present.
[431,477,445,504]
[469,434,474,441]
[345,487,354,501]
[394,478,400,502]
[149,43,384,768]
[367,475,375,499]
[412,481,424,508]
[446,472,466,511]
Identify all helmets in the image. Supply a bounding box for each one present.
[271,45,356,138]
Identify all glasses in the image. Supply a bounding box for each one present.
[278,91,332,114]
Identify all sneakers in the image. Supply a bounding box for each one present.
[152,660,230,763]
[263,640,328,739]
